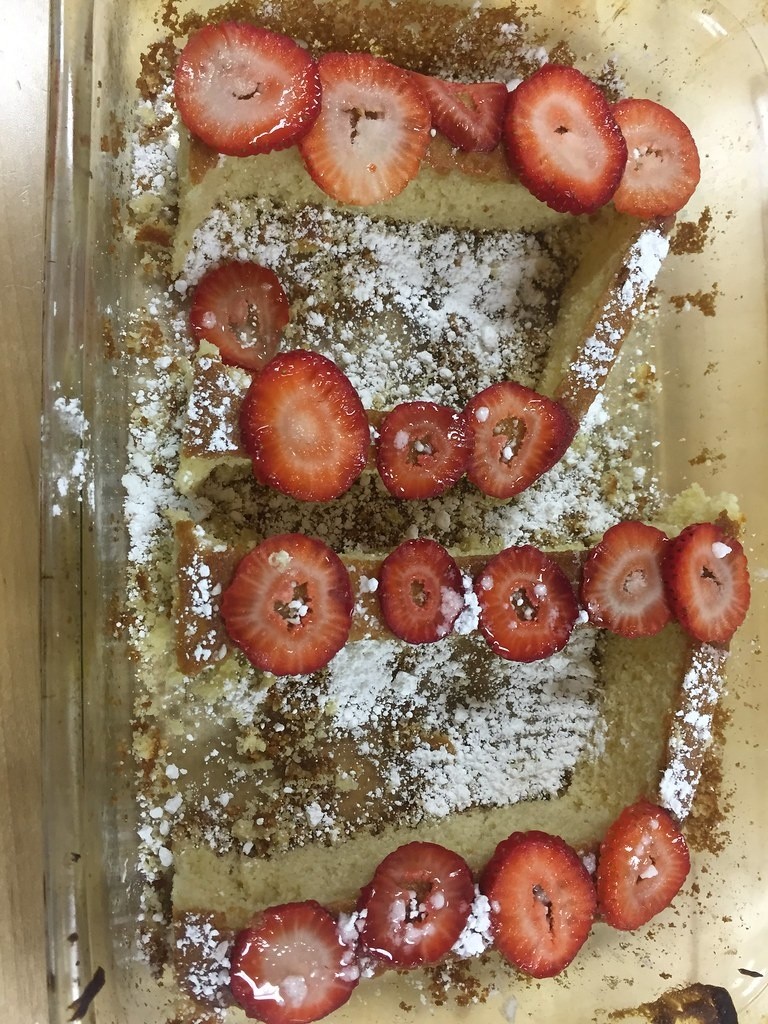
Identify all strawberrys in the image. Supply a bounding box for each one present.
[167,21,750,1023]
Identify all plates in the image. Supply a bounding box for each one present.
[34,1,765,1024]
